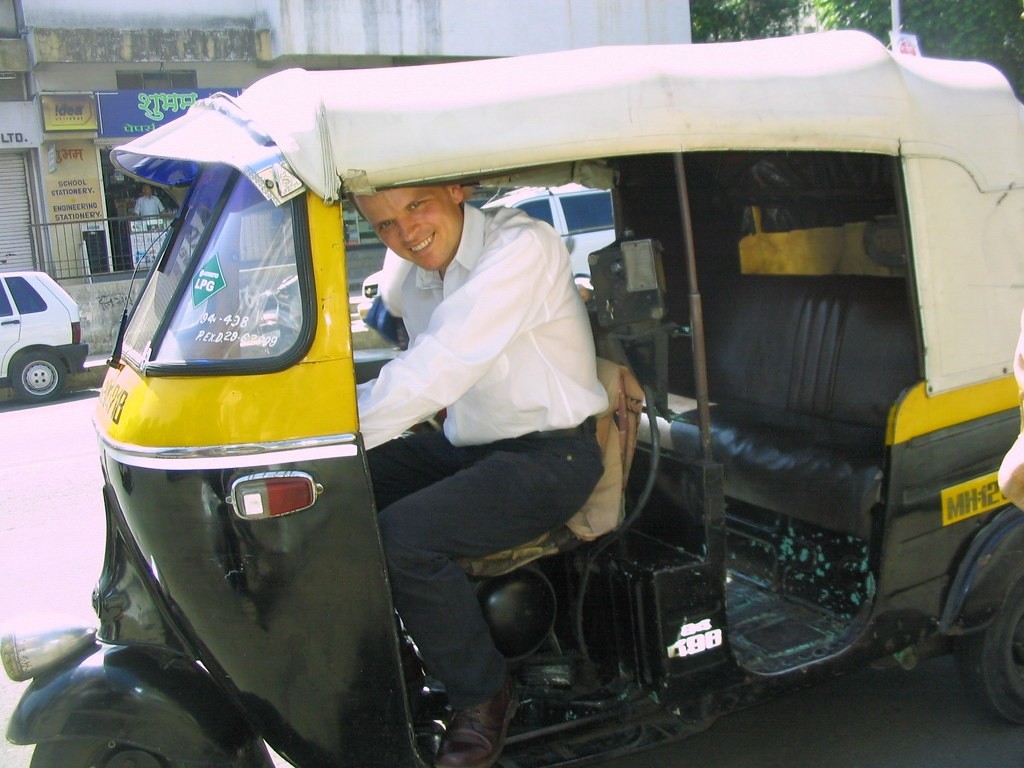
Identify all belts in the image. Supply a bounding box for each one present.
[524,416,598,440]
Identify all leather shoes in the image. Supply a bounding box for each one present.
[432,671,518,768]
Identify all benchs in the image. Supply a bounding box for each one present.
[669,266,921,539]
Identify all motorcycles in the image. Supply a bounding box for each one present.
[0,27,1023,768]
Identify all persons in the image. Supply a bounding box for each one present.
[133,183,167,220]
[349,181,611,768]
[997,308,1024,515]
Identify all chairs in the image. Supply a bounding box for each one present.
[454,355,660,710]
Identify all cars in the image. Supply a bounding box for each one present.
[360,183,617,337]
[0,270,89,404]
